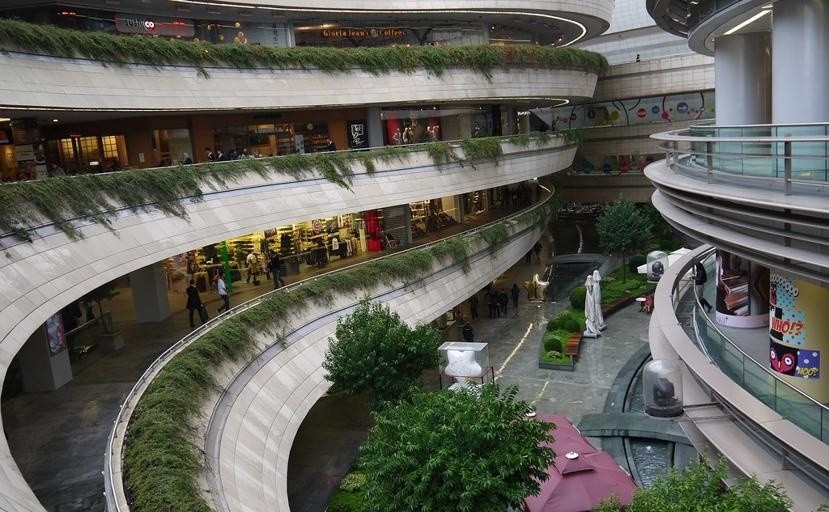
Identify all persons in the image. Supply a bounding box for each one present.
[499,183,542,209]
[640,292,653,313]
[526,250,532,264]
[186,280,206,328]
[180,146,264,165]
[692,262,712,313]
[323,138,336,151]
[534,242,543,260]
[269,249,285,289]
[216,271,229,315]
[392,126,440,145]
[244,249,258,284]
[458,283,520,342]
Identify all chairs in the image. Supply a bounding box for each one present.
[244,282,248,284]
[190,325,195,327]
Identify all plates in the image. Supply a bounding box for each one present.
[225,218,329,265]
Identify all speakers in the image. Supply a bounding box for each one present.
[699,300,704,309]
[702,299,712,312]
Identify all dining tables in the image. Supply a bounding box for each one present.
[83,281,124,350]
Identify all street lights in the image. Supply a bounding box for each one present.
[199,307,208,321]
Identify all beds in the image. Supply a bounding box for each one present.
[524,451,638,512]
[523,412,599,456]
